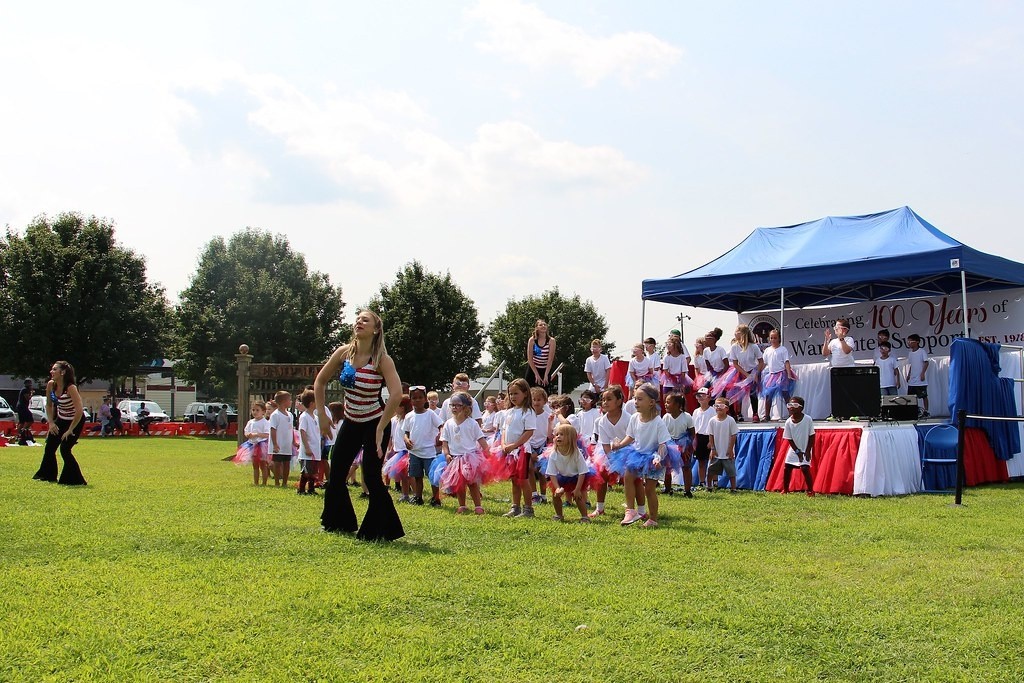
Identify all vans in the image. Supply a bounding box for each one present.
[28,395,91,422]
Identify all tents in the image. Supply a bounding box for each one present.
[641,206,1024,348]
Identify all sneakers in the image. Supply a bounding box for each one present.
[922,412,930,418]
[733,414,791,422]
[269,475,814,529]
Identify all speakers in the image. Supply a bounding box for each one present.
[880,395,918,421]
[830,365,881,419]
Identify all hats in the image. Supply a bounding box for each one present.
[670,330,681,336]
[694,387,712,397]
[639,382,659,400]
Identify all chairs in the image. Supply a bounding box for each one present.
[918,424,965,494]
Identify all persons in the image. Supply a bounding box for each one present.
[33,361,87,485]
[204,405,228,438]
[99,400,125,437]
[137,403,154,435]
[15,379,34,433]
[383,373,739,528]
[525,320,555,390]
[585,339,612,404]
[906,334,931,418]
[781,397,816,496]
[314,309,405,541]
[872,330,901,419]
[626,325,799,422]
[822,320,859,421]
[232,386,369,498]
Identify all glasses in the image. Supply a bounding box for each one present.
[452,382,468,387]
[408,386,426,399]
[496,399,505,405]
[449,404,463,410]
[712,404,728,410]
[908,341,916,344]
[696,394,709,399]
[786,403,803,408]
[484,402,495,407]
[427,400,438,405]
[589,334,714,354]
[833,326,850,330]
[879,336,888,339]
[881,347,889,351]
[578,398,593,403]
[735,332,741,335]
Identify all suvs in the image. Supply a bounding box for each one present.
[116,400,170,422]
[183,402,238,422]
[0,396,16,421]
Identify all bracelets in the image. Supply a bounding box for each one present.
[795,449,800,454]
[593,383,596,386]
